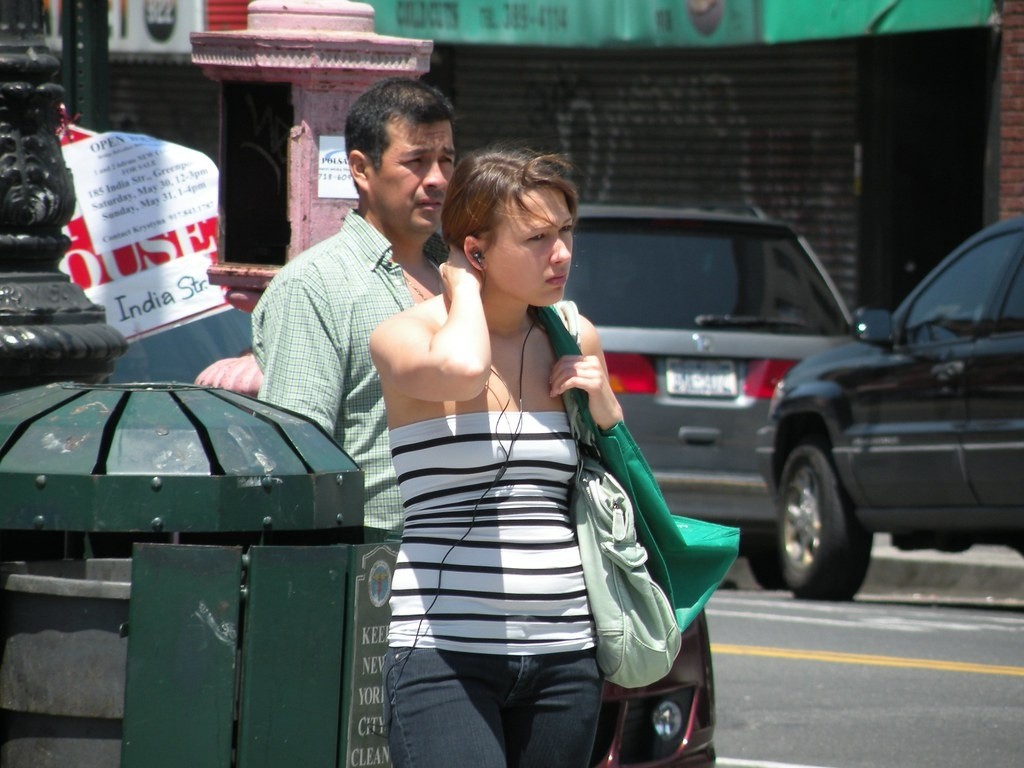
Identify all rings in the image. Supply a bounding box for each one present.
[577,355,586,362]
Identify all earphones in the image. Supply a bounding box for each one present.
[472,251,484,269]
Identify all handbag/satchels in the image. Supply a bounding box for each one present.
[528,303,741,689]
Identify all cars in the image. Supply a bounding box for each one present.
[756,217,1023,602]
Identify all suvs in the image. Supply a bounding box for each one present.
[425,201,864,596]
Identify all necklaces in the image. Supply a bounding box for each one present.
[405,256,442,301]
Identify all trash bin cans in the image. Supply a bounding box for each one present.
[0,380,403,768]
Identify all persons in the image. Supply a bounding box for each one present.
[252,78,457,541]
[369,146,624,768]
